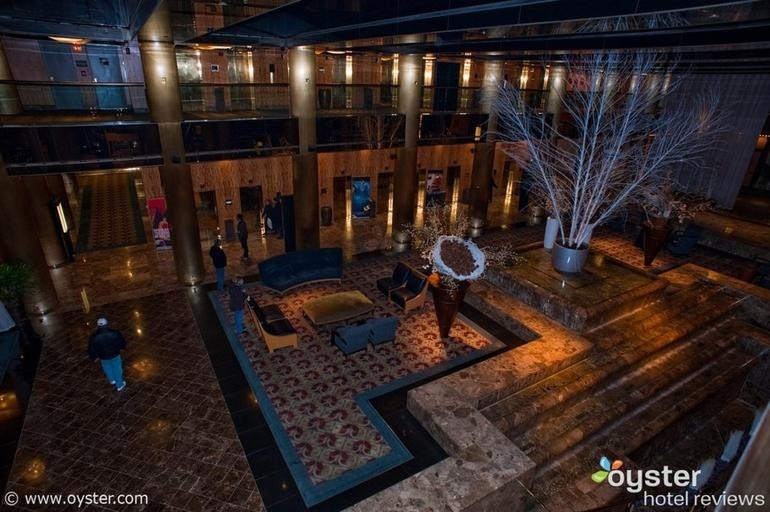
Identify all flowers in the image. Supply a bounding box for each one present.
[399,194,529,301]
[638,183,716,224]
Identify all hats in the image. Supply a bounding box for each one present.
[97,317,108,327]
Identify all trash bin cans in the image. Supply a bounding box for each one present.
[463,188,471,204]
[225,220,234,242]
[321,207,332,226]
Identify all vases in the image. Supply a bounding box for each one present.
[642,216,672,267]
[427,270,471,338]
[544,216,560,253]
[552,237,590,276]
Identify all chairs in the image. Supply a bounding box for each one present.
[244,294,297,354]
[376,259,430,314]
[330,315,399,358]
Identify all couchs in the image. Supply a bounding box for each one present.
[257,246,343,294]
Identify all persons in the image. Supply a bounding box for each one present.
[446,161,458,187]
[229,276,250,336]
[272,199,283,239]
[235,214,251,260]
[261,199,274,235]
[87,317,127,391]
[487,173,497,203]
[209,239,227,293]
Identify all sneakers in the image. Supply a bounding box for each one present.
[111,380,126,392]
